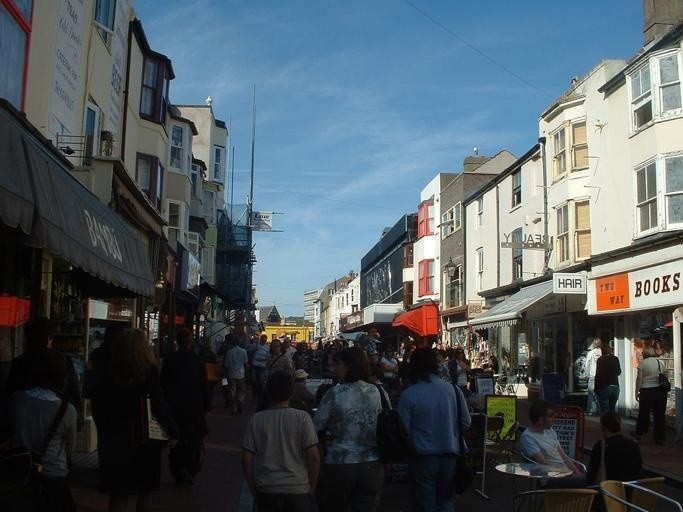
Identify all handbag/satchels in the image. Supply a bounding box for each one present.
[659,373,671,392]
[0,461,41,512]
[455,455,476,497]
[587,467,606,485]
[377,410,412,463]
[144,397,177,441]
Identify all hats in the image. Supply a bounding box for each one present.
[295,369,308,378]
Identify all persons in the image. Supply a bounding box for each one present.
[1,318,683,512]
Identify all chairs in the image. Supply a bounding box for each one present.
[601,477,683,511]
[520,451,560,489]
[494,420,519,467]
[589,476,669,512]
[292,399,315,414]
[509,484,598,512]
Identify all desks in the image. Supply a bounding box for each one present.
[494,458,575,503]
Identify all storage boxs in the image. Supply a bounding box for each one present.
[89,298,110,318]
